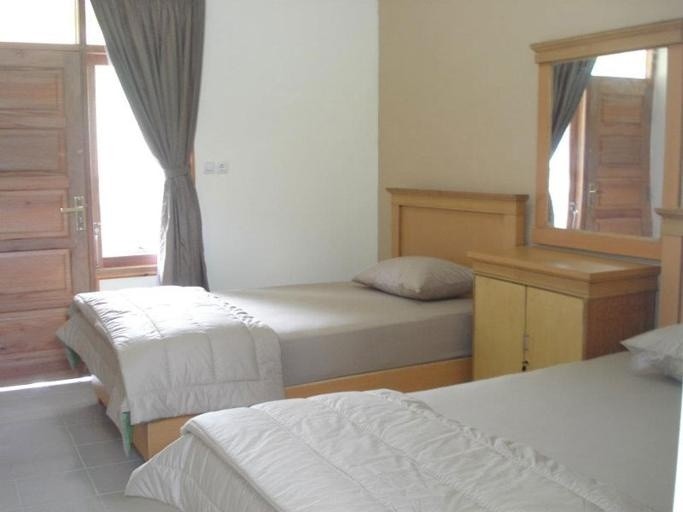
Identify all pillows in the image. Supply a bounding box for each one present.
[617,320,682,386]
[351,253,470,300]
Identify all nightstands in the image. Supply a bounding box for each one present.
[471,244,662,383]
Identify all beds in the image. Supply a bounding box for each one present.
[187,207,682,511]
[96,188,527,463]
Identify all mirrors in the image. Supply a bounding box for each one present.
[531,17,682,260]
